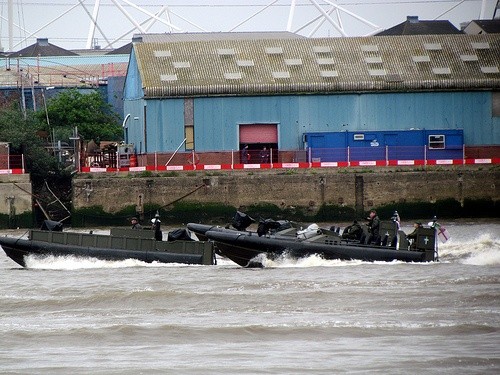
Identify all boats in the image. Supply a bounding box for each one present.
[0,210,217,268]
[187,211,438,268]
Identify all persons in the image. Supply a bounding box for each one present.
[366,210,380,235]
[259,147,270,163]
[241,144,250,164]
[406,221,423,251]
[131,217,142,230]
